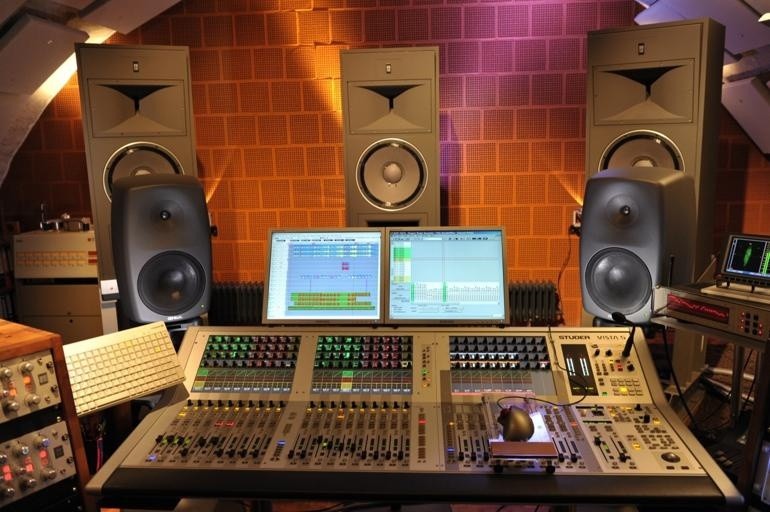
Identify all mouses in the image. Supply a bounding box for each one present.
[498,405,534,442]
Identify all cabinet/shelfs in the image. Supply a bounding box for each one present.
[15,276,102,347]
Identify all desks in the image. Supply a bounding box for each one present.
[650,315,770,417]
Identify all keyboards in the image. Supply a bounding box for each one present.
[62,321,186,417]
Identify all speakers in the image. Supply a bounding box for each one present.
[585,17,726,283]
[111,174,211,324]
[74,41,197,301]
[579,166,696,326]
[339,47,442,227]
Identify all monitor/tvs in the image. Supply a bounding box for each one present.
[261,227,385,325]
[384,226,510,325]
